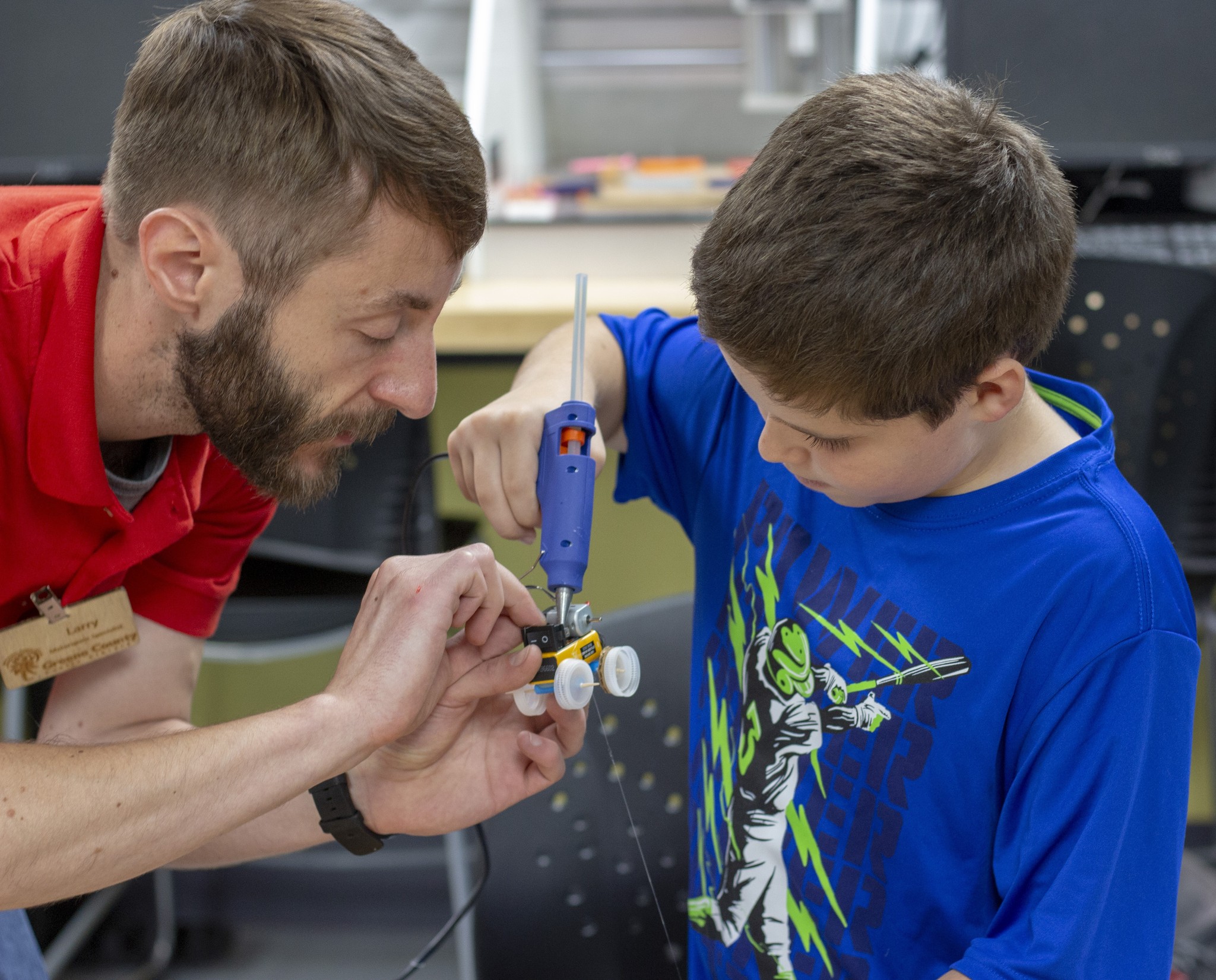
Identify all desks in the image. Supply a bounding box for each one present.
[187,224,703,726]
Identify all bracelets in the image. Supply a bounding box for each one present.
[309,774,386,855]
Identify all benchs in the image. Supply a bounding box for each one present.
[444,595,702,980]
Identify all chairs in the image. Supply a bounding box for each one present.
[38,410,451,980]
[1036,248,1216,850]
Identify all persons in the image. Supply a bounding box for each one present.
[449,60,1198,977]
[2,0,604,979]
